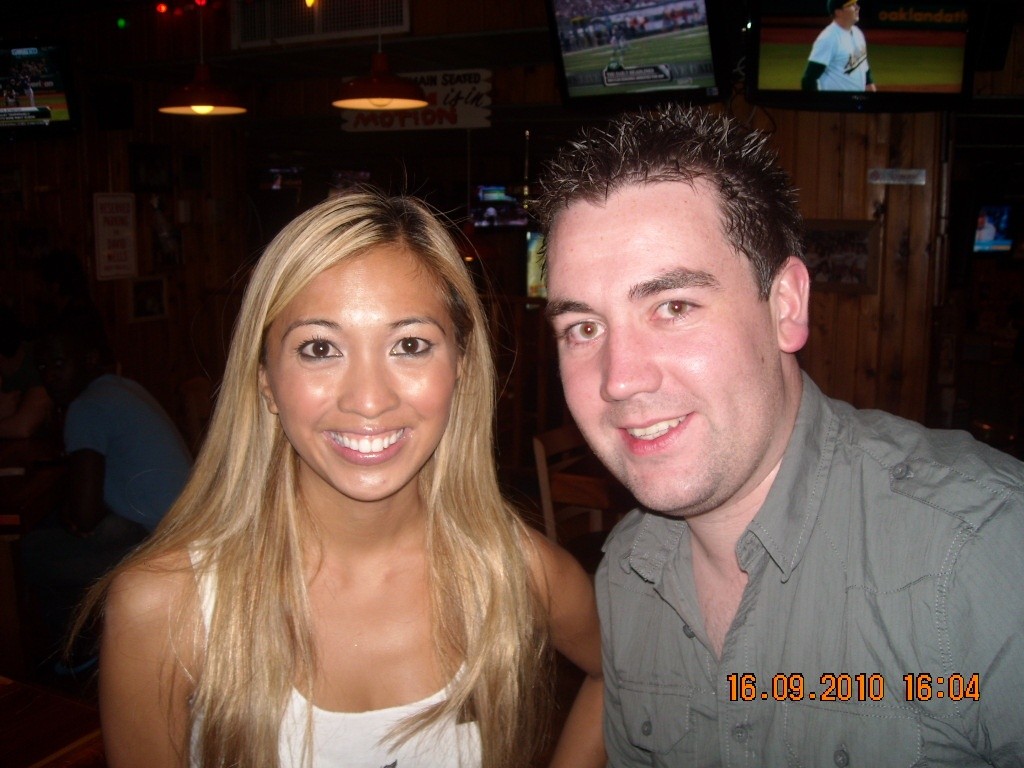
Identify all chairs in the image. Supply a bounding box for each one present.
[532,419,644,547]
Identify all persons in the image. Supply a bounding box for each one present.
[1,57,49,106]
[536,99,1024,768]
[555,0,705,69]
[71,185,610,768]
[2,248,199,700]
[802,0,878,93]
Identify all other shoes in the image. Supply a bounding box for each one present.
[56,642,101,675]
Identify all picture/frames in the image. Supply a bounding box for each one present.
[801,219,880,295]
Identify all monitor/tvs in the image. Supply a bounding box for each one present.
[545,0,731,115]
[0,33,86,146]
[747,0,978,114]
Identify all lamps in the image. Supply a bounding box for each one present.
[159,0,248,117]
[330,0,429,113]
